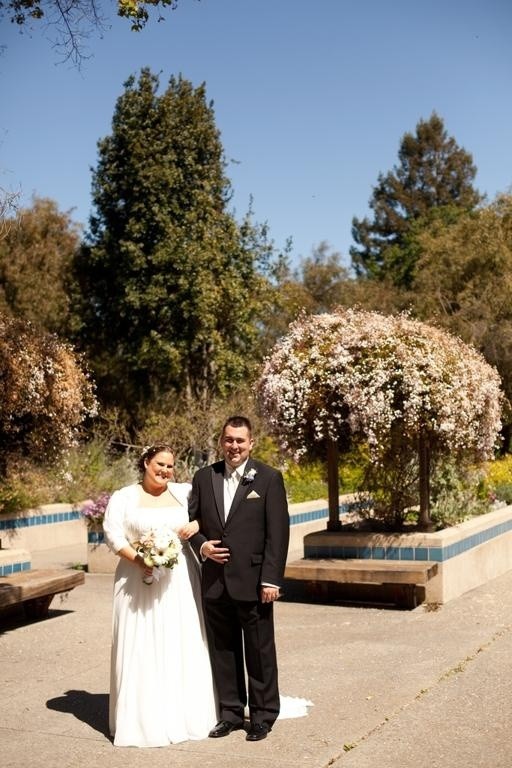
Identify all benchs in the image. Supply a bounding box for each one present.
[284,559,438,611]
[0,568,85,619]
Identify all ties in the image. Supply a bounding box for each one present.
[229,471,237,505]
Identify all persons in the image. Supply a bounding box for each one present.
[186,414,290,743]
[101,443,201,744]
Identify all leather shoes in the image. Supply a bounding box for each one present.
[210,721,243,737]
[246,725,268,740]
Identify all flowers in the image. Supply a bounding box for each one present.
[135,525,183,584]
